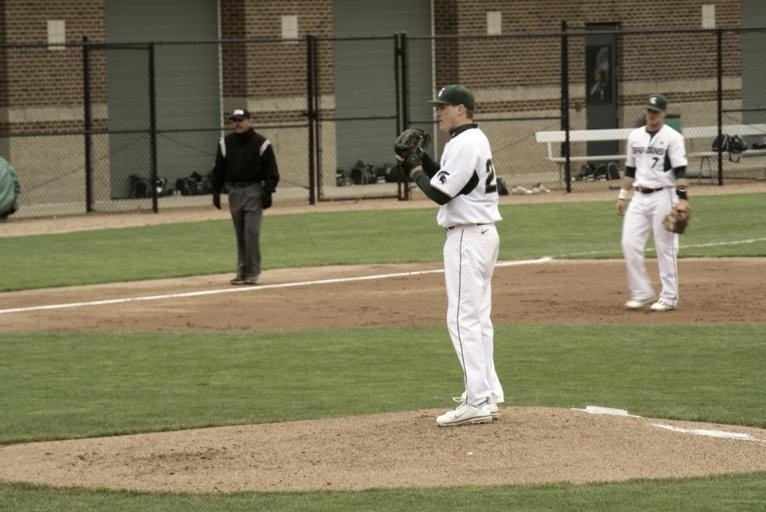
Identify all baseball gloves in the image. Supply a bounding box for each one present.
[394,129,432,160]
[660,201,691,234]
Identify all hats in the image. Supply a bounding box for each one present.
[642,94,669,113]
[424,84,476,110]
[228,109,251,120]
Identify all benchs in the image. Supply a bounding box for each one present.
[533,121,766,192]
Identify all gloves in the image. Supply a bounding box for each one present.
[211,193,221,209]
[261,190,272,210]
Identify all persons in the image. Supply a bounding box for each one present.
[616,93,689,312]
[393,84,505,428]
[211,108,279,286]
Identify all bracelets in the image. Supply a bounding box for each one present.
[676,185,688,200]
[618,188,630,200]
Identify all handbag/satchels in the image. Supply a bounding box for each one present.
[175,171,212,196]
[579,160,622,181]
[711,133,747,151]
[130,177,168,198]
[336,159,401,186]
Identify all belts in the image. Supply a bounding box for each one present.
[635,186,663,193]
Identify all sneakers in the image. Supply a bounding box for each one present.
[510,184,550,195]
[436,396,501,428]
[649,300,674,311]
[230,275,259,285]
[624,298,655,308]
